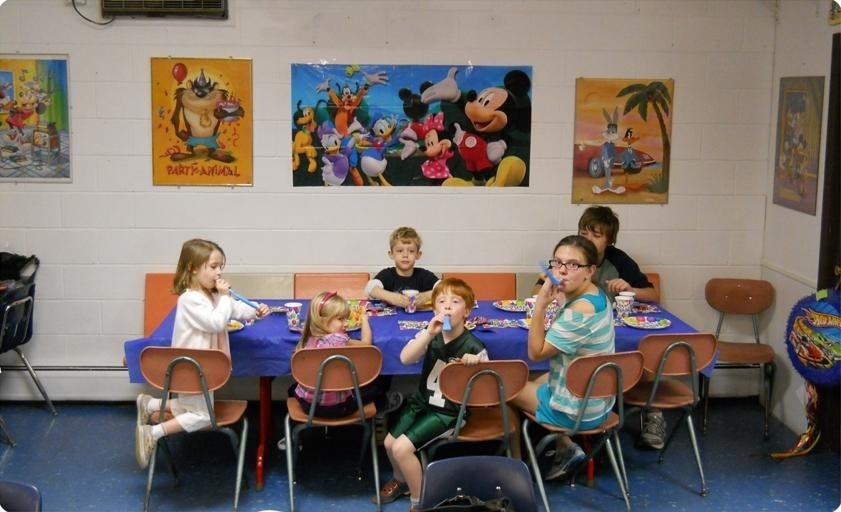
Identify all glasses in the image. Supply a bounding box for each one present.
[549,260,588,270]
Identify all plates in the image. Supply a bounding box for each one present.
[226,320,244,334]
[624,315,672,331]
[494,299,531,312]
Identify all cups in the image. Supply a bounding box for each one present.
[613,291,638,320]
[526,293,557,328]
[402,289,419,314]
[283,302,303,331]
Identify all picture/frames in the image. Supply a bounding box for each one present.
[0,51,73,183]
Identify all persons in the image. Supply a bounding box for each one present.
[134,236,270,469]
[371,278,492,512]
[531,206,670,450]
[502,234,617,485]
[277,291,404,453]
[365,225,442,310]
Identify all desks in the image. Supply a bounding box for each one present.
[124,298,724,493]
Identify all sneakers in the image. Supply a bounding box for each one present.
[545,443,585,480]
[136,425,156,468]
[278,436,303,451]
[641,416,667,449]
[384,391,403,413]
[372,478,410,503]
[136,393,152,426]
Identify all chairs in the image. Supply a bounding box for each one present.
[1,249,60,449]
[700,278,775,436]
